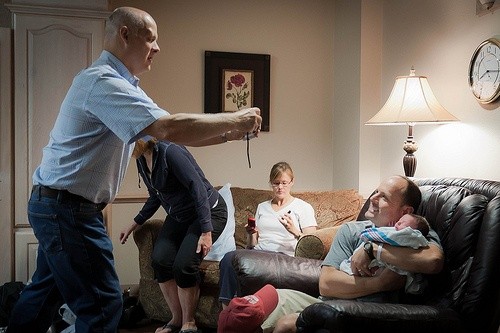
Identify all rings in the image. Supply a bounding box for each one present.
[358,269,362,272]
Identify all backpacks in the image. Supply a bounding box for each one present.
[0,281,26,327]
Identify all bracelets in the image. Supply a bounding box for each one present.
[222,133,231,142]
[295,231,303,240]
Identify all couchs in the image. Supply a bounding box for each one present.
[235,177,500,333]
[134,186,363,327]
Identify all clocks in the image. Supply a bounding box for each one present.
[468,37,500,105]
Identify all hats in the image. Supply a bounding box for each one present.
[217,284,278,333]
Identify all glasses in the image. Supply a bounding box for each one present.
[269,179,293,188]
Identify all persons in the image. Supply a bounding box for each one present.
[339,213,431,276]
[217,284,279,333]
[218,162,317,310]
[261,175,443,333]
[119,135,229,333]
[7,6,263,333]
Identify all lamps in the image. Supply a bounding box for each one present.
[364,68,458,177]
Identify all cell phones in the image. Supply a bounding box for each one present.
[248,217,256,228]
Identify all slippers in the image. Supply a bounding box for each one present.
[155,324,181,333]
[180,325,198,333]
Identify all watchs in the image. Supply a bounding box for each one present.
[363,241,375,260]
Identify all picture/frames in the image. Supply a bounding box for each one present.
[205,51,272,132]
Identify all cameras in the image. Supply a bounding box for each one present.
[244,127,259,141]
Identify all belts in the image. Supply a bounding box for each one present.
[32,185,94,205]
